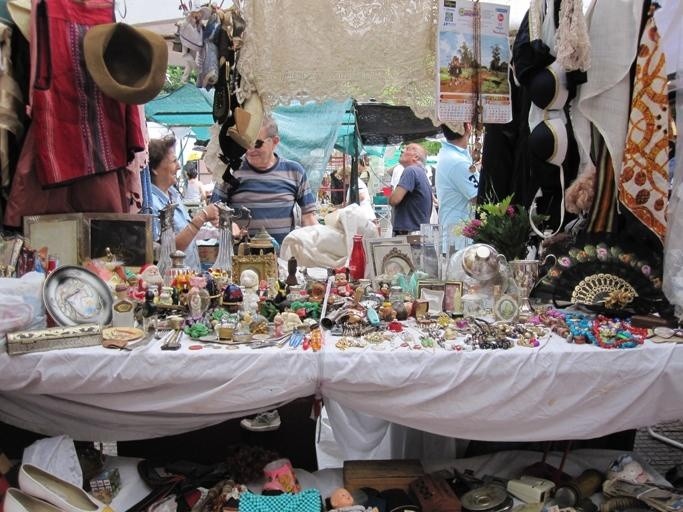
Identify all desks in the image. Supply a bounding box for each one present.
[1,321,682,467]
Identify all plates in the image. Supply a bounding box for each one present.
[41,266,114,329]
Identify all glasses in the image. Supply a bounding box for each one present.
[254,137,273,149]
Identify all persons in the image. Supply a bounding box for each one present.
[435,121,481,256]
[327,488,378,511]
[144,110,320,275]
[337,143,432,238]
[137,264,163,296]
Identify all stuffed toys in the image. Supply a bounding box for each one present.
[333,266,353,297]
[378,281,391,300]
[239,265,260,310]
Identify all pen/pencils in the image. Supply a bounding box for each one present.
[159,329,183,344]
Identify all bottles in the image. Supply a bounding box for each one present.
[349,234,367,279]
[448,244,510,307]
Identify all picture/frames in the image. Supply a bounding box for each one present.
[23,212,152,274]
[231,255,274,286]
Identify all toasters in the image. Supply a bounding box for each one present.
[390,285,404,316]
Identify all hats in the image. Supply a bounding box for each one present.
[82,23,168,104]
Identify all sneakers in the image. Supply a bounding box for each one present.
[240,409,283,432]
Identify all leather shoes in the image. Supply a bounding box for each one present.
[2,464,112,512]
